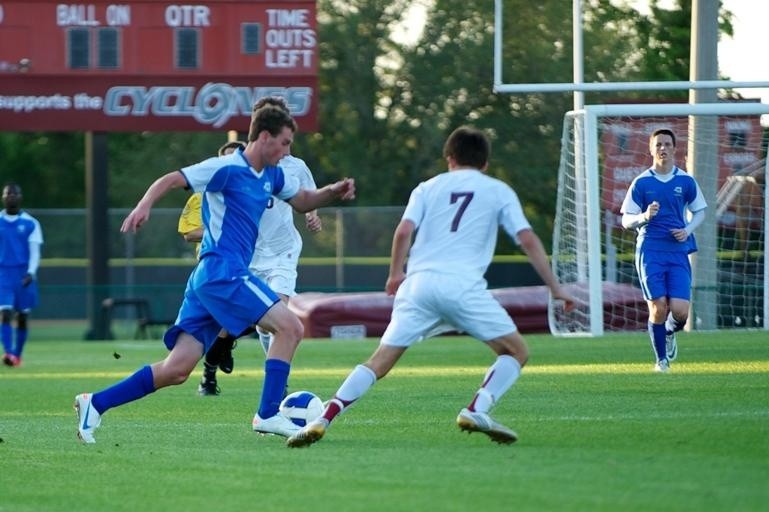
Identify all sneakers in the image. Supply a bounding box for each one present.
[73,392,103,446]
[284,417,328,448]
[456,407,517,446]
[252,411,302,437]
[197,339,234,395]
[665,330,677,361]
[655,359,668,371]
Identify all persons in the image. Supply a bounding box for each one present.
[286,125,581,450]
[230,98,323,402]
[621,127,707,374]
[180,140,245,397]
[0,180,43,371]
[73,108,356,448]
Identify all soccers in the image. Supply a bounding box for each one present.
[279,391,323,427]
